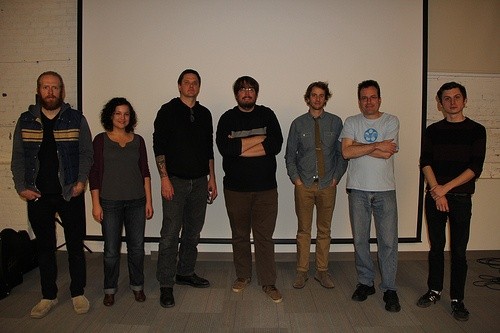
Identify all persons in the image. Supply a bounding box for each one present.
[417,81,488,318]
[335,80,405,311]
[149,68,217,308]
[284,82,349,285]
[89,95,155,305]
[214,75,283,303]
[10,70,95,319]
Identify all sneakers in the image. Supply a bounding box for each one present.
[314,270,335,289]
[29,297,58,318]
[131,288,146,302]
[260,284,283,303]
[415,290,440,307]
[351,283,375,300]
[160,286,175,308]
[71,295,90,313]
[173,273,210,288]
[230,278,250,292]
[450,301,469,322]
[103,293,114,307]
[292,270,308,289]
[381,290,401,312]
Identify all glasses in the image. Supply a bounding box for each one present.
[359,95,380,101]
[236,88,255,93]
